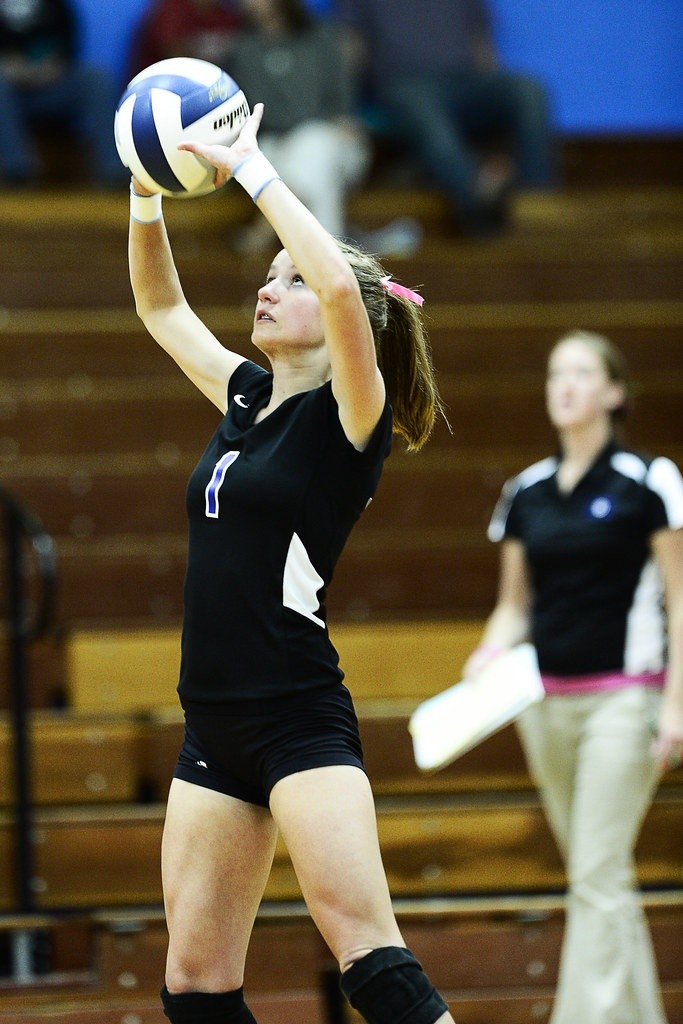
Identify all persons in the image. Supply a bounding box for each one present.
[0,1,568,262]
[122,97,457,1024]
[460,325,681,1021]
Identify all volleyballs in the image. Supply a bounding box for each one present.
[111,55,252,204]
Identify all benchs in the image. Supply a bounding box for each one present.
[0,197,682,1022]
